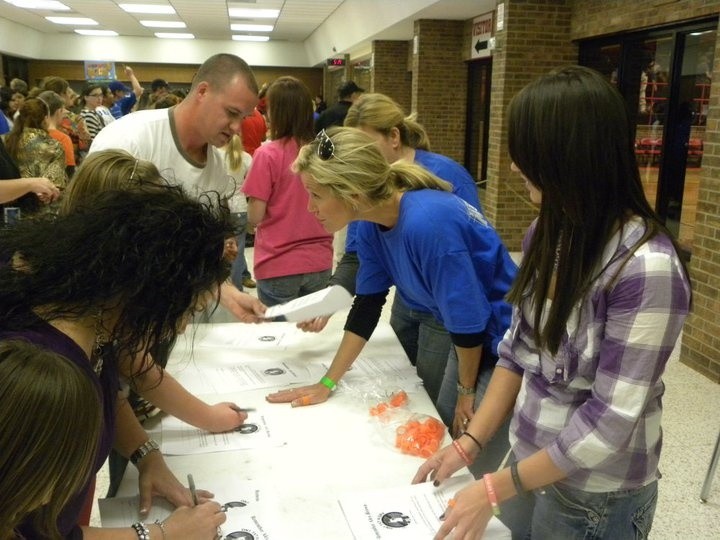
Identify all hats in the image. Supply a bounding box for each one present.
[153,79,170,89]
[337,80,365,95]
[110,81,131,91]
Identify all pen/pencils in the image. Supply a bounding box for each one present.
[234,407,257,414]
[187,472,200,507]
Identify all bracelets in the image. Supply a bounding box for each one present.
[482,473,502,516]
[461,431,483,452]
[155,518,167,539]
[319,376,337,392]
[456,382,477,395]
[510,459,533,501]
[452,439,475,465]
[129,438,161,465]
[130,521,151,539]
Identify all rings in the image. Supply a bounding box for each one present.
[215,525,223,540]
[290,388,295,394]
[302,396,311,406]
[446,499,456,507]
[463,418,469,427]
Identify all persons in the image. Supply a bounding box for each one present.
[1,192,230,540]
[267,128,530,467]
[410,66,693,540]
[294,90,486,405]
[0,338,104,540]
[58,51,270,327]
[0,150,251,435]
[228,79,339,327]
[0,61,368,231]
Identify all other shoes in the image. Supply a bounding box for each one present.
[245,232,254,247]
[242,276,255,287]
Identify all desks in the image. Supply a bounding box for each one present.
[100,310,520,539]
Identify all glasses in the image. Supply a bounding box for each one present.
[86,93,104,98]
[316,128,349,165]
